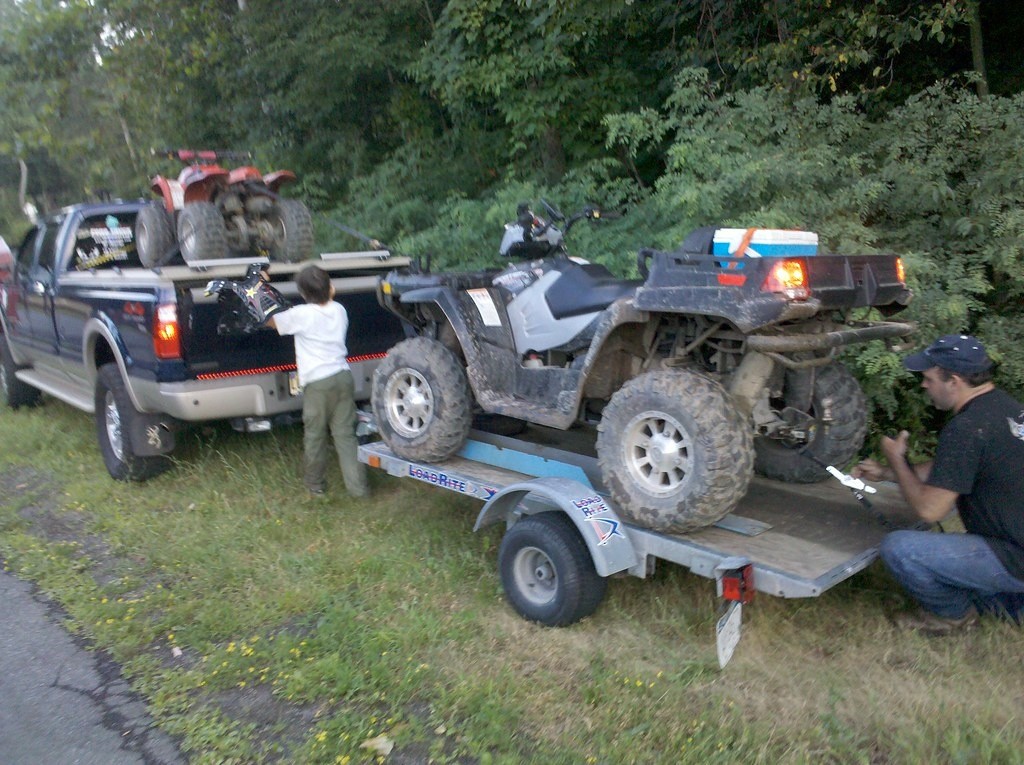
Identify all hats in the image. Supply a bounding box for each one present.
[904,335,993,374]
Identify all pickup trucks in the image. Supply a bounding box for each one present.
[0,194,425,481]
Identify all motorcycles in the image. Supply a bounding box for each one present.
[367,194,918,534]
[133,147,318,264]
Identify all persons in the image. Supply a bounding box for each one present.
[259,265,369,498]
[850,335,1024,634]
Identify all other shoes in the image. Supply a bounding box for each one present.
[301,478,328,493]
[890,606,983,637]
[342,489,374,506]
